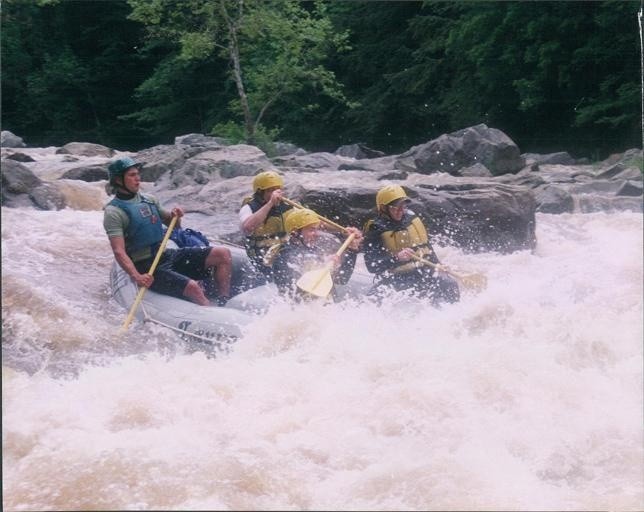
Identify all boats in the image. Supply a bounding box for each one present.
[104,235,374,351]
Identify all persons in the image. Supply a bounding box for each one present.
[239,172,326,281]
[272,208,362,308]
[364,185,460,308]
[103,157,231,306]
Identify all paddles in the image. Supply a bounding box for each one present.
[297,234,354,298]
[411,254,485,295]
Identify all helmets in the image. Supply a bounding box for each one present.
[376,184,412,212]
[284,209,322,237]
[253,171,285,195]
[108,157,149,185]
